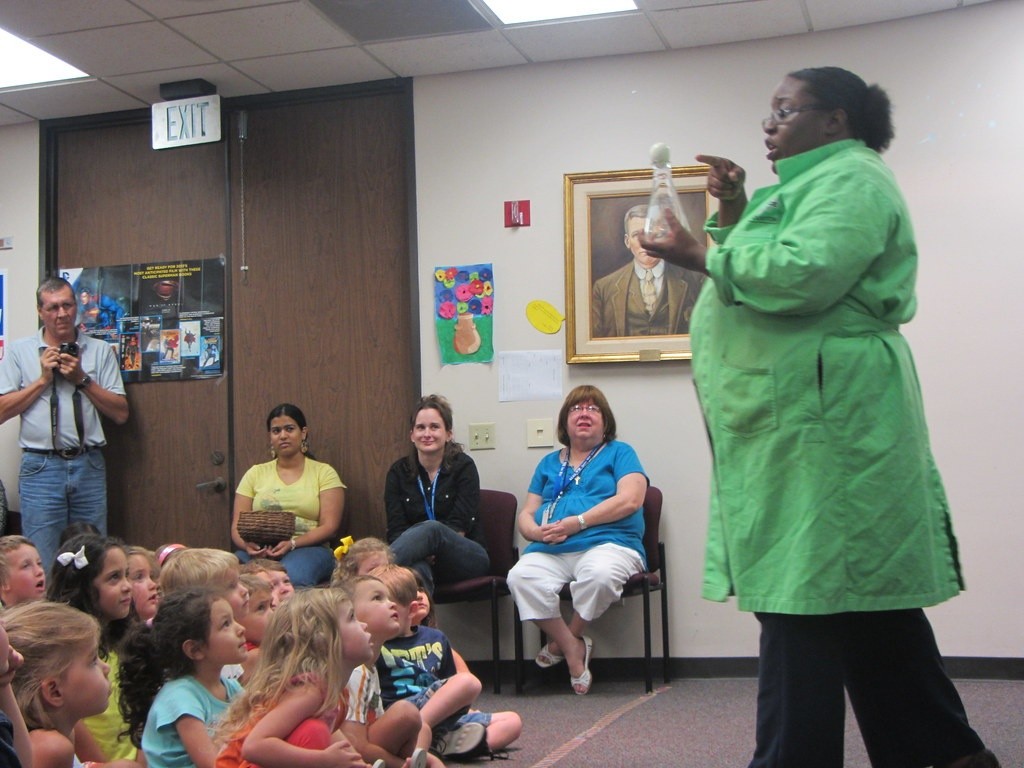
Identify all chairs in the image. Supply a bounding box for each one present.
[415,490,521,694]
[511,486,670,694]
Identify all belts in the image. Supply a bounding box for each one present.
[24,445,100,456]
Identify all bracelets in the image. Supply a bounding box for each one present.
[578,515,587,530]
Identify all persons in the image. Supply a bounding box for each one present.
[637,66,1001,768]
[506,385,650,695]
[384,394,490,596]
[0,277,129,577]
[231,403,348,586]
[0,522,522,768]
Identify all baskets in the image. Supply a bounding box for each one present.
[238,510,296,544]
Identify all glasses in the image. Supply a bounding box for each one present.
[567,404,602,414]
[763,102,825,127]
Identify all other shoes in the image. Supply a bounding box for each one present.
[429,721,488,760]
[535,644,566,667]
[372,759,386,768]
[402,748,428,768]
[570,636,593,695]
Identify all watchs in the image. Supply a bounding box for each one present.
[78,376,90,390]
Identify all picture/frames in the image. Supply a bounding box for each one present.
[565,163,714,365]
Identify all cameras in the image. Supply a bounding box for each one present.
[57,342,80,359]
